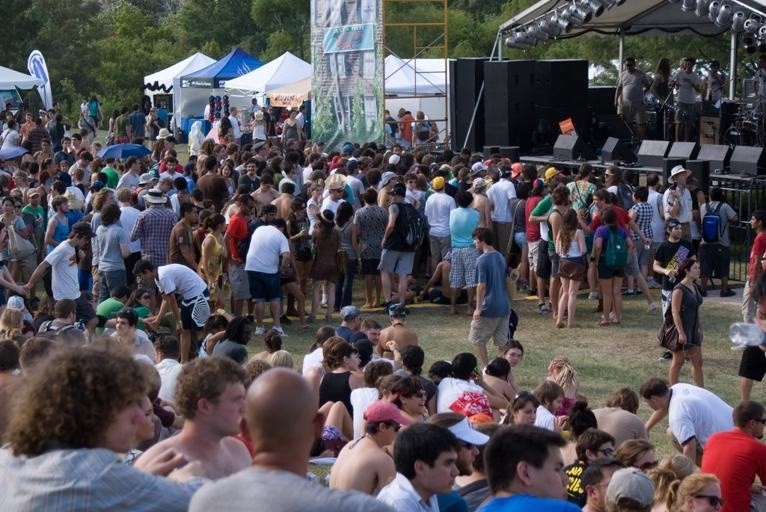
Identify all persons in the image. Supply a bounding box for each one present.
[649,58,675,128]
[612,57,653,141]
[668,57,687,87]
[667,57,703,142]
[752,54,766,102]
[3,93,766,510]
[704,63,726,101]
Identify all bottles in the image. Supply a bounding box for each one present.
[729,322,766,349]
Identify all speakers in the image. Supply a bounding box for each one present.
[449,56,510,153]
[482,59,537,156]
[636,139,672,167]
[499,146,521,162]
[696,144,733,174]
[600,136,621,165]
[535,59,588,156]
[662,157,688,193]
[667,141,701,168]
[483,145,499,155]
[686,160,709,196]
[552,134,581,162]
[729,145,766,176]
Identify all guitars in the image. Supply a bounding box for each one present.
[643,90,689,118]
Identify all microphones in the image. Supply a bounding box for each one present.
[681,66,688,73]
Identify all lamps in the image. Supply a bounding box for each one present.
[499,0,766,52]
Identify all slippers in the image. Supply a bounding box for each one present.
[612,319,620,325]
[599,320,608,325]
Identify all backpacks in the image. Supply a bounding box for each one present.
[702,213,725,245]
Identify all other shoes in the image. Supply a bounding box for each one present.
[720,289,733,297]
[253,299,328,337]
[528,289,552,314]
[588,289,641,299]
[699,287,708,297]
[647,303,656,314]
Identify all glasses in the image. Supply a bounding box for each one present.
[140,295,152,300]
[512,390,536,410]
[752,418,766,424]
[598,449,616,456]
[690,492,726,510]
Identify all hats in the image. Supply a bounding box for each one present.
[69,220,98,239]
[7,294,29,314]
[606,467,656,510]
[3,95,729,228]
[361,401,416,427]
[445,416,490,448]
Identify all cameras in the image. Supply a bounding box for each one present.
[670,181,678,190]
